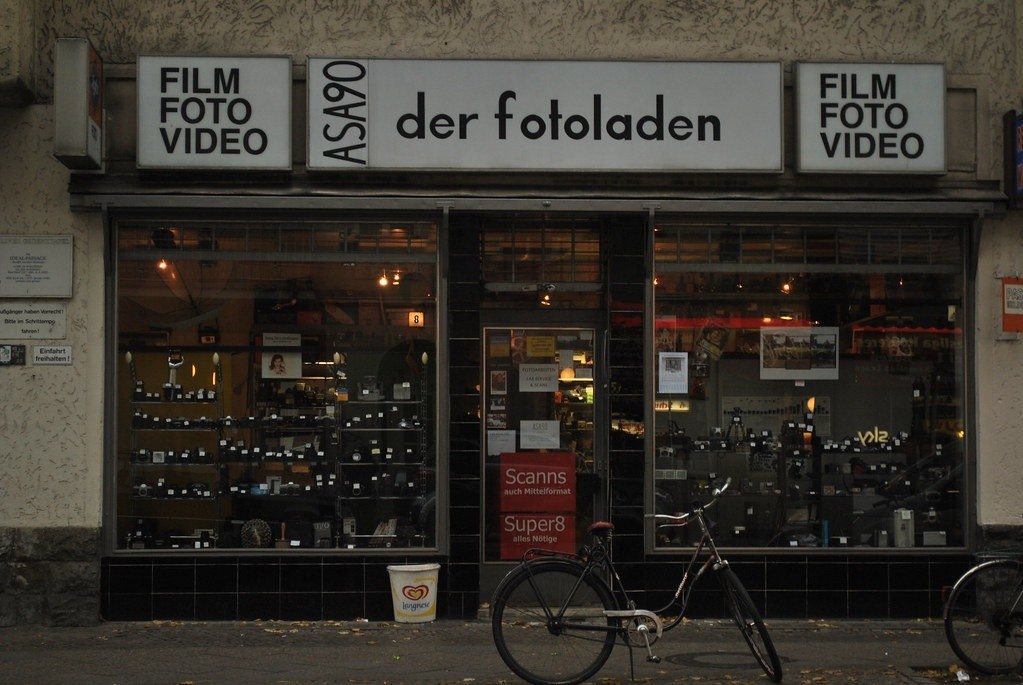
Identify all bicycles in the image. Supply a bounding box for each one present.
[766,489,962,549]
[942,548,1023,675]
[489,477,782,685]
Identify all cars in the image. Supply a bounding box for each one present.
[768,341,832,360]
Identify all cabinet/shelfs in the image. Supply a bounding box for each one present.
[129,353,428,549]
[656,441,924,544]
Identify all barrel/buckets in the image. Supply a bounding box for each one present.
[387,563,440,623]
